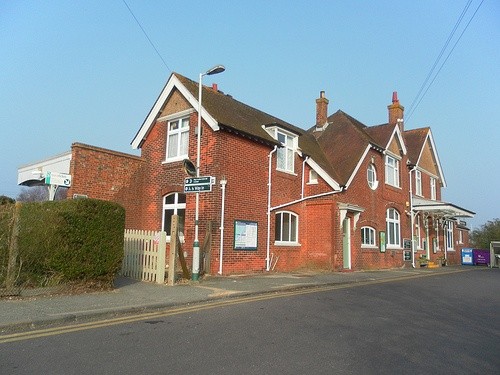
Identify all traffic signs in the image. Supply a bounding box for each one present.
[183,177,212,192]
[44,171,73,188]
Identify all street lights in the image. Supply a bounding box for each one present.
[191,65,227,276]
